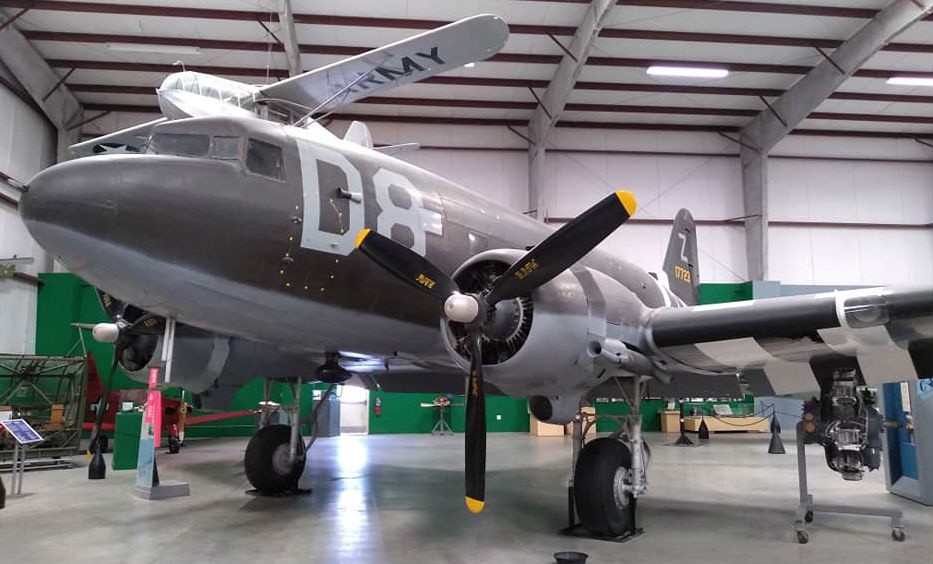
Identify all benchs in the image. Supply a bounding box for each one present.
[82,390,186,447]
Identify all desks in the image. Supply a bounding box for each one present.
[684,415,769,440]
[420,402,464,436]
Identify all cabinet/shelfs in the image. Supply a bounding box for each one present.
[256,410,279,430]
[528,403,597,438]
[660,411,681,433]
[311,397,341,437]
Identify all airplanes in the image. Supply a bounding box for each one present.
[82,351,262,454]
[20,13,932,539]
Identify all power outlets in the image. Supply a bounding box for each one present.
[496,414,501,420]
